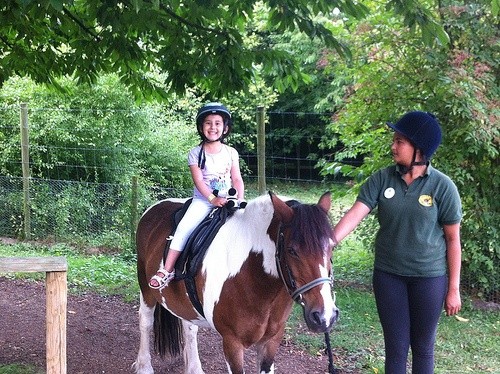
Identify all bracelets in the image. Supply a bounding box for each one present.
[208,194,214,202]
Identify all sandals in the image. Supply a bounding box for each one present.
[148,267,175,289]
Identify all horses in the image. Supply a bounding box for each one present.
[129,190,340,374]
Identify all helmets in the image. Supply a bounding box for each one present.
[386,112,442,157]
[196,101,232,133]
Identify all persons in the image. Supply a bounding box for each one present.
[326,112,462,374]
[147,101,245,290]
